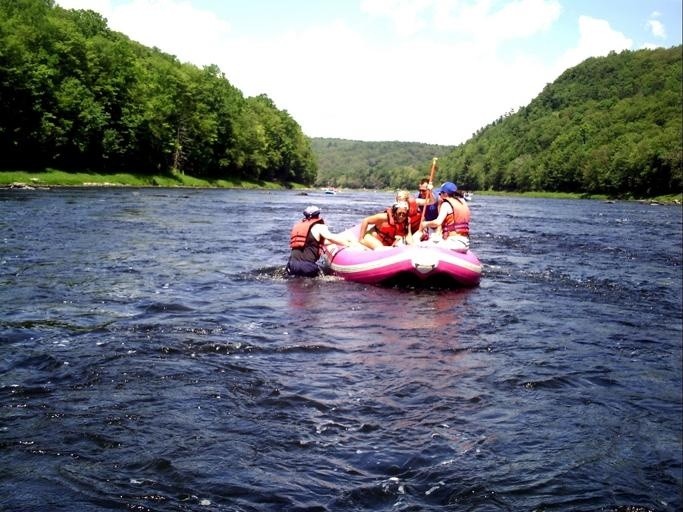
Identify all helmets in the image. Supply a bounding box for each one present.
[303,205,319,216]
[392,191,409,210]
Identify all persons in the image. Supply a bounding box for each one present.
[287,206,351,278]
[359,179,470,254]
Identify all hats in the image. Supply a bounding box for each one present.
[432,182,456,194]
[396,208,407,215]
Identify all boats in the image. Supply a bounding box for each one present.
[325,191,335,194]
[463,194,471,200]
[326,223,482,285]
